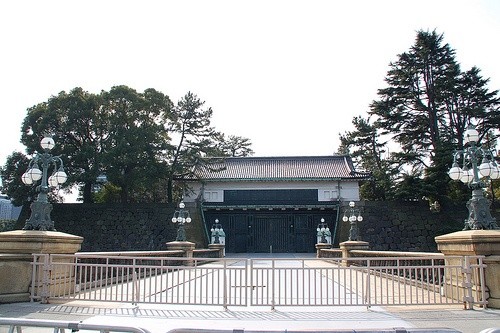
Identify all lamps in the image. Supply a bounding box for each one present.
[430,201,440,214]
